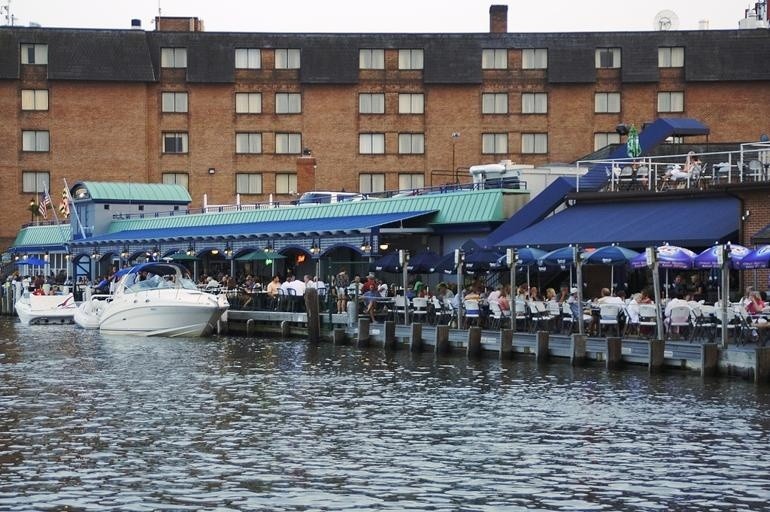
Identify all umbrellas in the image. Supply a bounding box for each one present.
[168,252,202,261]
[627,121,642,167]
[13,255,48,267]
[235,249,287,261]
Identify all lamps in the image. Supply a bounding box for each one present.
[13,236,388,263]
[208,168,215,174]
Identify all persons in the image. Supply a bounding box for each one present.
[6,271,120,301]
[407,272,769,330]
[677,151,702,189]
[670,155,701,189]
[134,268,396,325]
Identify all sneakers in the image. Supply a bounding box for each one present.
[363,309,379,323]
[337,311,346,313]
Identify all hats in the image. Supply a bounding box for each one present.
[436,281,449,288]
[570,287,578,295]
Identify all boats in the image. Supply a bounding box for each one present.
[15,262,230,338]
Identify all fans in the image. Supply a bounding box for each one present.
[390,220,418,242]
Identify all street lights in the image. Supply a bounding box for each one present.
[31,198,35,221]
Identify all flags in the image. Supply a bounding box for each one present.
[58,185,72,218]
[38,185,50,219]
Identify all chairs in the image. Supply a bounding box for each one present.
[64,283,87,301]
[605,159,770,193]
[206,283,769,349]
